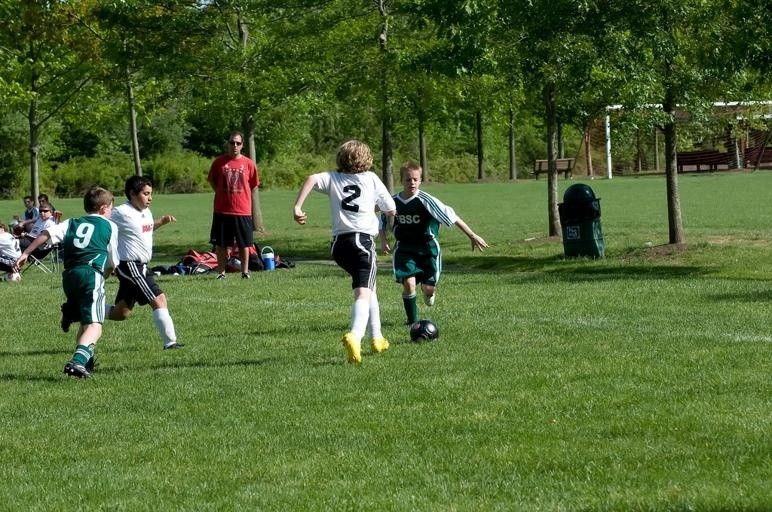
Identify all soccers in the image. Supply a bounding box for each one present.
[410,319,439,343]
[3,272,23,282]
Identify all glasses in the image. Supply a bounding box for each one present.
[229,141,241,146]
[39,210,49,213]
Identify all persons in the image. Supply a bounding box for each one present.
[59,175,185,350]
[11,187,120,378]
[378,162,490,326]
[0,192,65,282]
[293,140,397,363]
[207,131,262,279]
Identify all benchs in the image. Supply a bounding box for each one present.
[530,158,576,180]
[676,146,772,173]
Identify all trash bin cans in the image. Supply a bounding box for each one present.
[557,184,604,260]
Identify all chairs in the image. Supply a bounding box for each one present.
[21,245,61,273]
[52,210,63,223]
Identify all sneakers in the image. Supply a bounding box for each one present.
[64,357,99,378]
[61,303,74,332]
[0,272,22,282]
[369,337,390,354]
[341,332,362,365]
[421,283,436,305]
[242,272,251,279]
[163,343,185,350]
[217,274,226,280]
